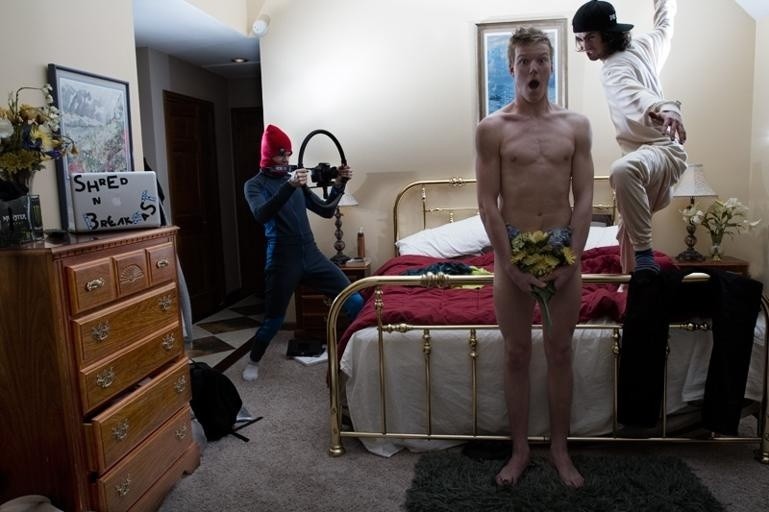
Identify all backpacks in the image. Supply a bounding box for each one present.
[189,360,263,441]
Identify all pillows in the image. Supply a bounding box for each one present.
[390,210,620,259]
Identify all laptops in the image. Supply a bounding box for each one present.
[69,170,162,235]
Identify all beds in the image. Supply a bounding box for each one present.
[327,174,769,463]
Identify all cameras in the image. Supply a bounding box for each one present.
[311,162,339,187]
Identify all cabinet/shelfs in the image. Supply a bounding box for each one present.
[0,224,204,511]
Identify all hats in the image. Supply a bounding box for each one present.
[572,0,634,35]
[260,125,292,172]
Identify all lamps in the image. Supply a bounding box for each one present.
[667,158,717,262]
[320,185,357,264]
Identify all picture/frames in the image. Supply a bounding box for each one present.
[49,60,135,234]
[474,16,568,122]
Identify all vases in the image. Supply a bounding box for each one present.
[710,235,723,260]
[0,192,45,249]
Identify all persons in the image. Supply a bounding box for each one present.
[242,121,363,383]
[475,25,595,490]
[570,0,689,295]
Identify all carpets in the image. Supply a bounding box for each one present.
[399,436,722,510]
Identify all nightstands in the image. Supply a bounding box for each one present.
[674,256,751,278]
[293,254,371,347]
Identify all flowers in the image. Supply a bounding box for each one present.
[690,195,763,252]
[493,217,576,328]
[0,77,80,203]
